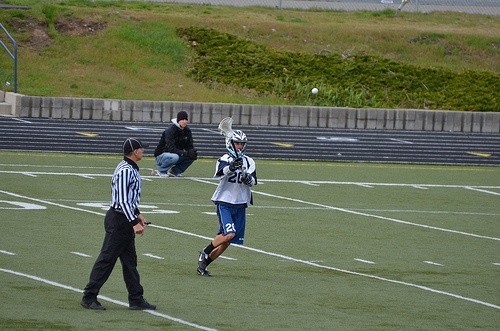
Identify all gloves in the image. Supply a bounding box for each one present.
[229,158,243,172]
[183,149,198,160]
[241,173,252,185]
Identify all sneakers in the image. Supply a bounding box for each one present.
[81,292,106,310]
[167,165,185,176]
[129,296,156,310]
[196,266,212,276]
[198,247,208,269]
[152,167,169,177]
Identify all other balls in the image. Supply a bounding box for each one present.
[312,87,318,95]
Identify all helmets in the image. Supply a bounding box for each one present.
[225,130,247,159]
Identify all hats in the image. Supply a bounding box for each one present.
[123,138,149,154]
[177,111,188,122]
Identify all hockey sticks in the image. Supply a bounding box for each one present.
[217,116,250,180]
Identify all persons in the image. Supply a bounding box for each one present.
[81,136,157,310]
[196,129,257,277]
[154,110,198,177]
